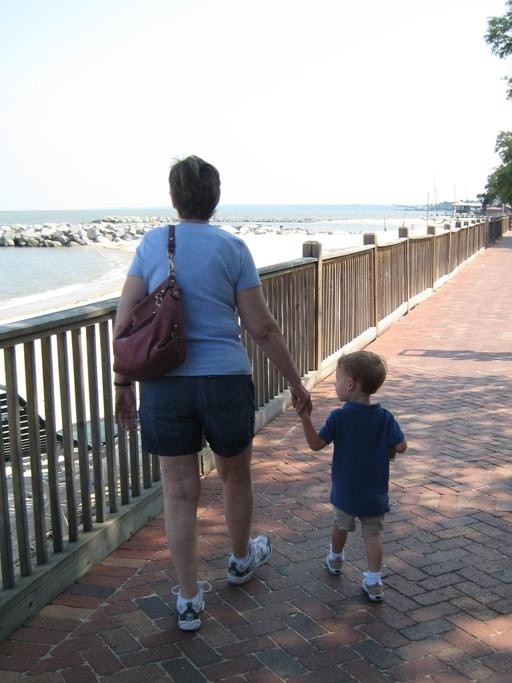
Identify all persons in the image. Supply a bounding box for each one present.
[295,349,407,602]
[113,156,312,632]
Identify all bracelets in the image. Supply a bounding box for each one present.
[113,381,134,389]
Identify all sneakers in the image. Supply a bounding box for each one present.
[226,535,272,585]
[326,552,345,575]
[171,582,212,631]
[361,577,385,602]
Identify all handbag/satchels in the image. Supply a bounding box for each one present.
[113,275,185,380]
